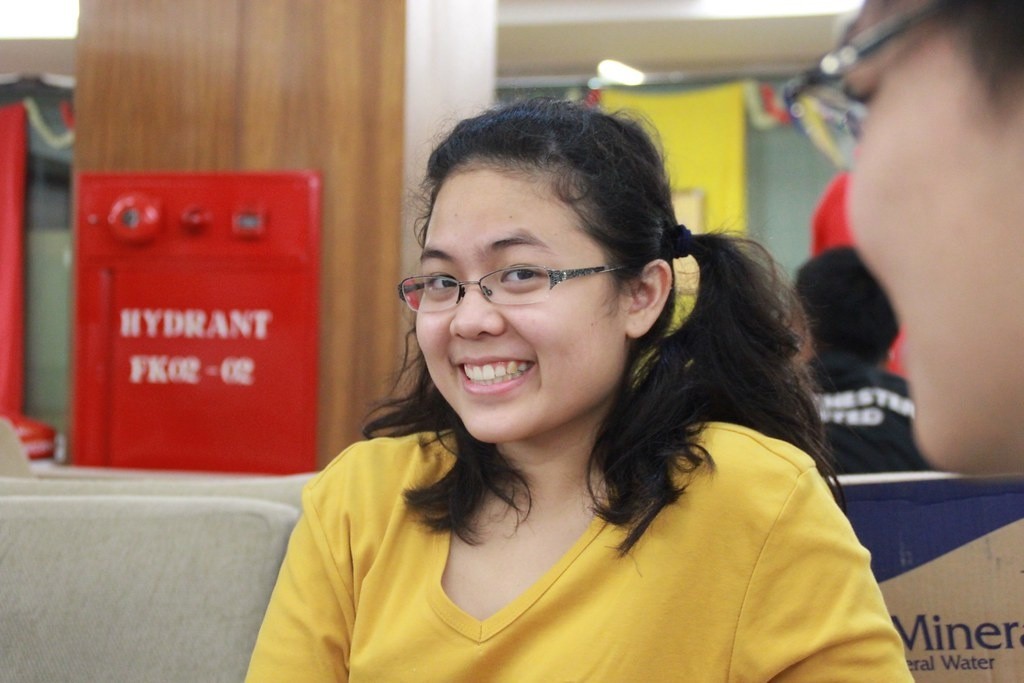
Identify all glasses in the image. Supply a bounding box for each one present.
[782,2,943,170]
[397,261,627,315]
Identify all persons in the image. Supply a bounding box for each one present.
[840,0,1024,480]
[244,98,917,683]
[791,246,931,475]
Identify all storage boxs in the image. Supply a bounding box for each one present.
[819,472,1024,682]
